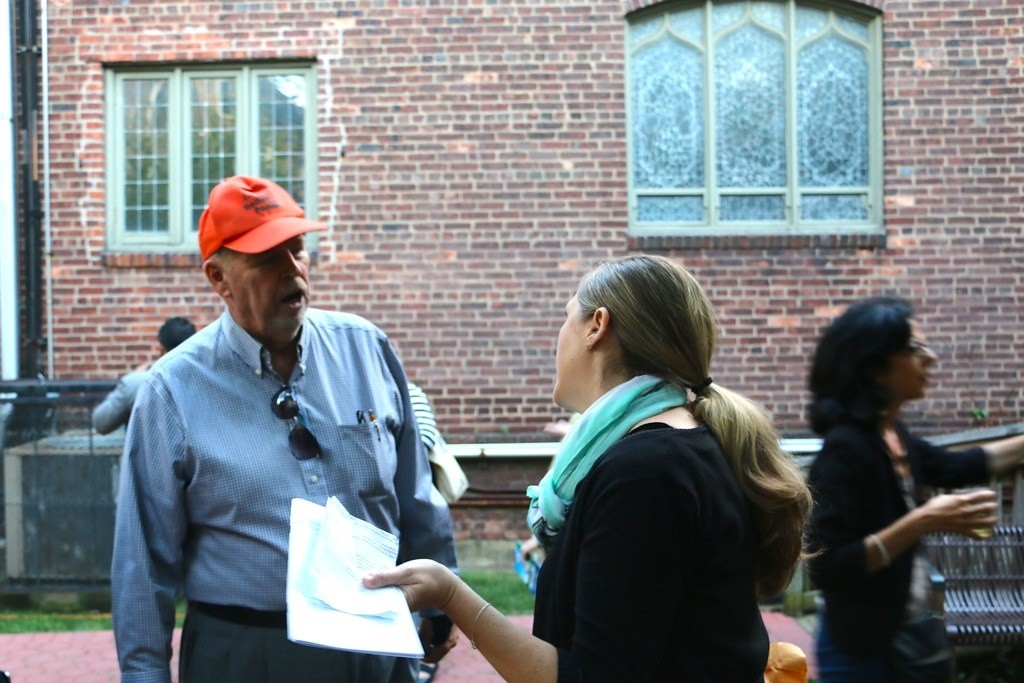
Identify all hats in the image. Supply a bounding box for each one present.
[198,176,327,264]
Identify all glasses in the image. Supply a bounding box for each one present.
[271,384,325,461]
[897,337,934,359]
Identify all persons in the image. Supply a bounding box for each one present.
[810,296,1024,683]
[111,176,458,683]
[363,254,811,683]
[91,318,197,434]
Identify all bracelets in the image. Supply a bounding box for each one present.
[471,603,491,649]
[870,534,891,569]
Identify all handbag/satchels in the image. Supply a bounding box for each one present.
[891,611,957,683]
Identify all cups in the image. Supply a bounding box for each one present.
[952,487,993,538]
